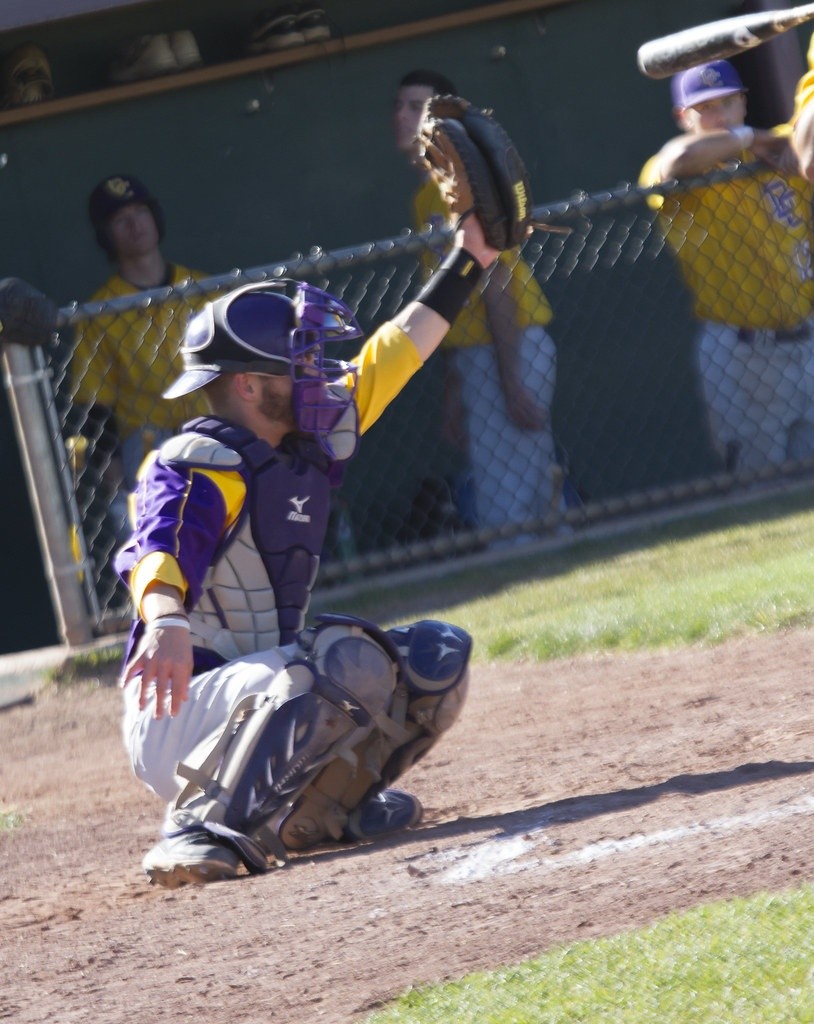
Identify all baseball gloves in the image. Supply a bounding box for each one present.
[415,95,533,251]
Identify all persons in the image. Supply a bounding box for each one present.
[394,71,574,560]
[115,96,536,887]
[640,36,814,482]
[75,170,230,526]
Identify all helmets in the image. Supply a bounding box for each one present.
[161,288,319,401]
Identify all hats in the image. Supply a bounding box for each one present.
[669,54,748,113]
[86,173,161,222]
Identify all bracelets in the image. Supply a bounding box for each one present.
[440,245,483,286]
[145,611,190,635]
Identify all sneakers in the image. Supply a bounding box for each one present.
[106,31,177,81]
[299,9,329,44]
[230,11,307,59]
[0,47,52,106]
[170,29,203,69]
[141,829,239,888]
[332,792,430,845]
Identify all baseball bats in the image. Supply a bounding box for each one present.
[636,2,814,97]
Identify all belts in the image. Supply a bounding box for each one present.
[736,325,814,346]
